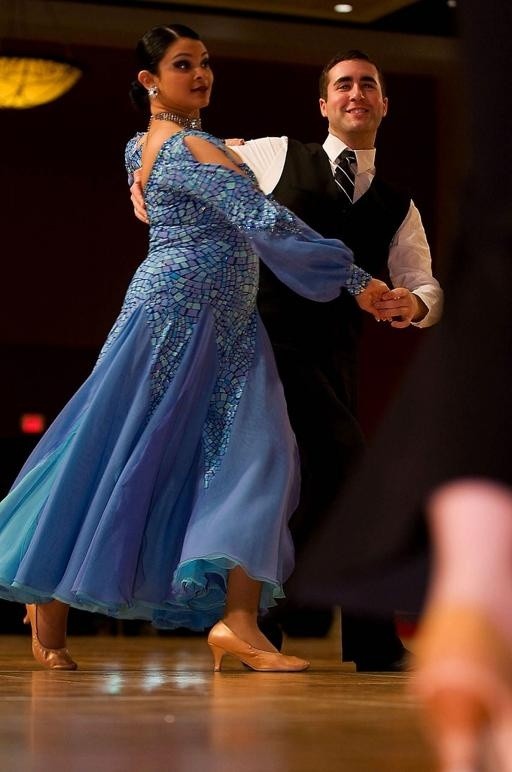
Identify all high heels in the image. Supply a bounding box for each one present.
[23,604,77,671]
[207,621,309,672]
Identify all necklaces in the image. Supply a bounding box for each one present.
[147,112,203,130]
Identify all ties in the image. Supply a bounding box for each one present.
[334,151,356,204]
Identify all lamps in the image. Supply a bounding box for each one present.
[0,52,85,111]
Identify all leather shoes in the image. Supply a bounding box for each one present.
[356,649,418,672]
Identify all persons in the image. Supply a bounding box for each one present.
[0,20,394,671]
[130,53,444,672]
